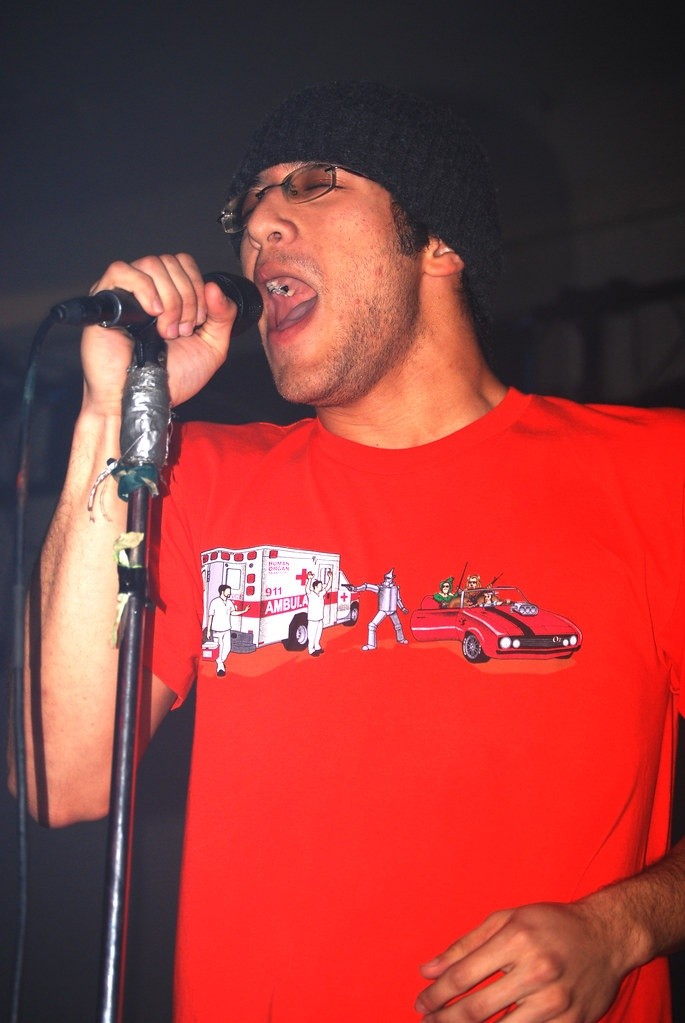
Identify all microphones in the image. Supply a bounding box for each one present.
[55,272,263,337]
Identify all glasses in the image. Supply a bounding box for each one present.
[216,162,371,235]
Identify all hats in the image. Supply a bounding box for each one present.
[235,89,540,315]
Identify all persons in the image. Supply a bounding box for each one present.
[1,81,685,1023]
